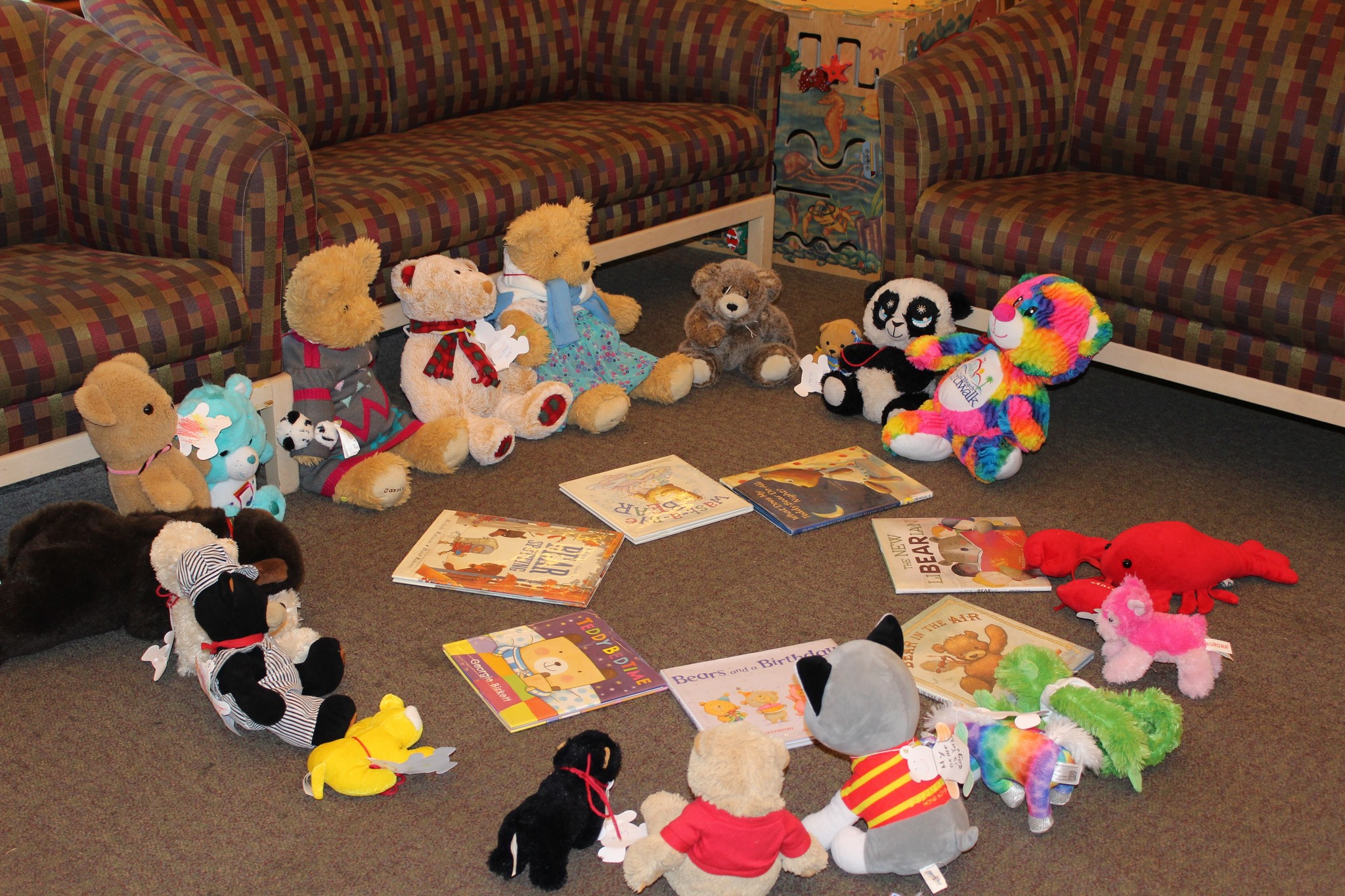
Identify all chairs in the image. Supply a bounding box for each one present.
[0,0,296,495]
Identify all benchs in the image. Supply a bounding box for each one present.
[876,0,1345,428]
[74,0,788,333]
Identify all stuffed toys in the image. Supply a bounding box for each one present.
[276,237,469,513]
[881,272,1113,483]
[70,351,211,513]
[388,254,576,466]
[174,541,356,745]
[794,613,979,875]
[679,257,800,391]
[972,644,1186,793]
[0,501,308,667]
[1020,519,1300,621]
[301,694,436,801]
[820,275,972,425]
[148,518,319,676]
[491,192,695,433]
[812,319,862,372]
[621,724,832,896]
[485,729,624,894]
[175,374,288,522]
[1077,576,1224,700]
[919,700,1103,833]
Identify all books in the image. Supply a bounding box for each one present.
[387,446,1096,754]
